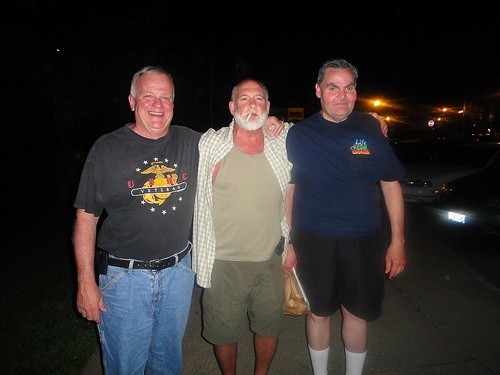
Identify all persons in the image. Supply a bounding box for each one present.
[73,67,285,375]
[283,57,407,375]
[191,80,388,375]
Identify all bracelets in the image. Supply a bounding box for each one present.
[288,240,293,244]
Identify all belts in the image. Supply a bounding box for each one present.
[107,243,192,270]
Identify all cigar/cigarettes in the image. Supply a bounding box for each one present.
[246,114,252,124]
